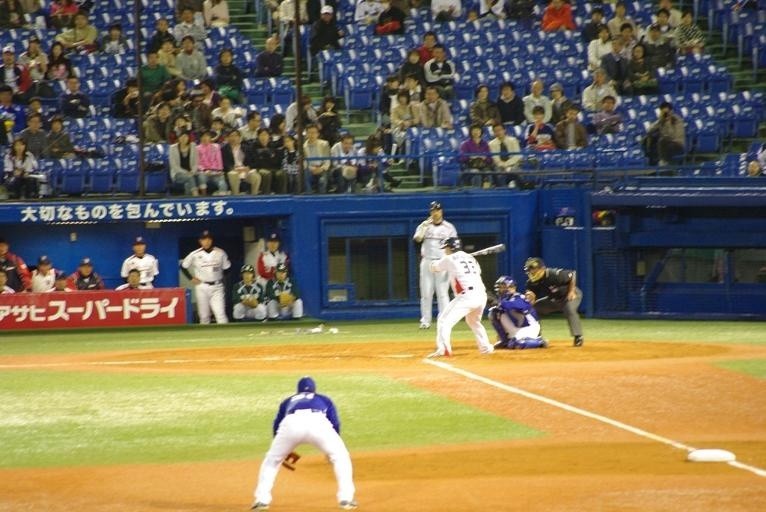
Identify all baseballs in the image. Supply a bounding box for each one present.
[288,457,294,463]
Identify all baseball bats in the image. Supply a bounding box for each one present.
[471,244,506,256]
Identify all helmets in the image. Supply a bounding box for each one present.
[241,233,289,273]
[298,377,314,390]
[430,201,546,296]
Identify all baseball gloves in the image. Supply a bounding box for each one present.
[283,453,300,472]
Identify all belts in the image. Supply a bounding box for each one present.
[204,282,214,285]
[140,283,146,286]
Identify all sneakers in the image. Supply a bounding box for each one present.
[428,353,450,357]
[250,501,269,510]
[339,500,357,510]
[575,335,583,346]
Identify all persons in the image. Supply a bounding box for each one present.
[249,376,358,511]
[518,254,587,347]
[423,234,496,360]
[411,198,459,331]
[0,0,765,199]
[1,218,307,326]
[486,272,549,350]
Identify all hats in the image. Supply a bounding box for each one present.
[79,257,90,265]
[198,230,212,238]
[40,255,49,264]
[133,236,145,245]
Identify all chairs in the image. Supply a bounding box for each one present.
[0,1,766,199]
